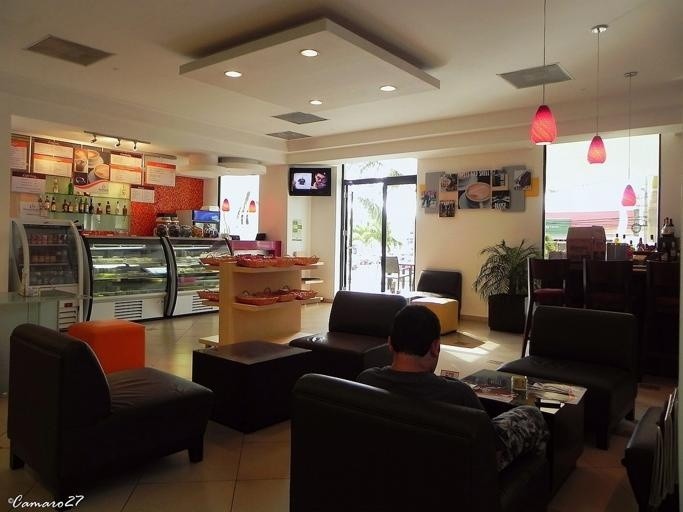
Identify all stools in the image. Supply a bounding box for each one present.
[411,295,459,336]
[70,318,148,375]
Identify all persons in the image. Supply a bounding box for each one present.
[352,303,552,479]
[312,171,327,189]
[421,174,458,217]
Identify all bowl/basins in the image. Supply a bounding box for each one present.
[464,182,491,202]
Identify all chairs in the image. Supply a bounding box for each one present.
[8,324,213,512]
[518,259,678,383]
[381,257,411,294]
[402,270,462,320]
[293,290,406,379]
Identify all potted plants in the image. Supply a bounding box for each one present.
[474,240,536,334]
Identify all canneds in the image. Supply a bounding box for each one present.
[28,232,68,244]
[509,374,528,400]
[29,249,68,264]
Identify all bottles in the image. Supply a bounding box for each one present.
[52,179,58,193]
[29,252,57,264]
[38,195,127,216]
[68,177,73,195]
[613,233,654,254]
[661,217,674,237]
[156,223,212,238]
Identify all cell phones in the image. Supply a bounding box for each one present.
[535,397,560,407]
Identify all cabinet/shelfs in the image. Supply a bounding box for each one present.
[45,192,130,217]
[195,263,326,346]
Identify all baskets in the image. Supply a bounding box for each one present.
[199,255,237,266]
[292,255,320,265]
[257,287,295,301]
[271,256,296,267]
[237,255,276,268]
[235,290,280,306]
[196,288,220,300]
[283,284,316,300]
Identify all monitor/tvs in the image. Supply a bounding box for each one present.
[288,167,332,197]
[255,233,265,240]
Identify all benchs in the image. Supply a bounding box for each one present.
[284,371,549,512]
[495,305,639,449]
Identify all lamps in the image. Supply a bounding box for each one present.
[531,1,638,210]
